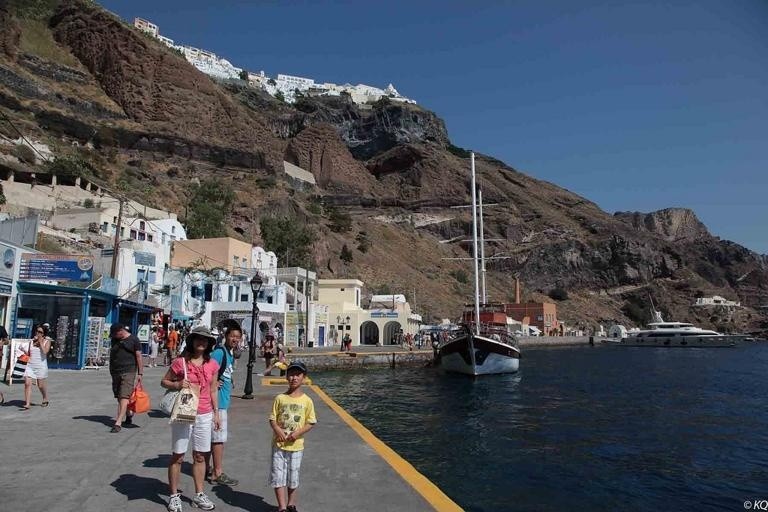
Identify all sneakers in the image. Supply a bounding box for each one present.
[206,467,240,486]
[287,504,298,512]
[110,425,121,432]
[191,492,215,509]
[125,411,134,424]
[167,494,182,512]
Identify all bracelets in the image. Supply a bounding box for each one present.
[213,407,219,412]
[138,374,143,376]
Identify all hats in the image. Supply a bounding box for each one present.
[109,323,122,337]
[186,325,216,352]
[287,360,307,371]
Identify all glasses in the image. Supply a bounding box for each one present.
[35,329,43,335]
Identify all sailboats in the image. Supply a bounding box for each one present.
[431,149,521,378]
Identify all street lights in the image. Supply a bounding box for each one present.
[253,307,259,362]
[335,315,352,351]
[417,321,421,349]
[239,270,262,400]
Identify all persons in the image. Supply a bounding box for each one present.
[109,321,143,432]
[343,333,352,350]
[258,332,284,377]
[148,326,190,367]
[209,323,242,486]
[269,359,316,511]
[393,328,451,349]
[19,325,51,409]
[0,325,11,406]
[161,325,224,512]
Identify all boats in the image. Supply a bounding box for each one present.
[619,293,749,349]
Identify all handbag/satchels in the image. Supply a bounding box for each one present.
[128,389,149,413]
[159,389,178,414]
[18,354,29,362]
